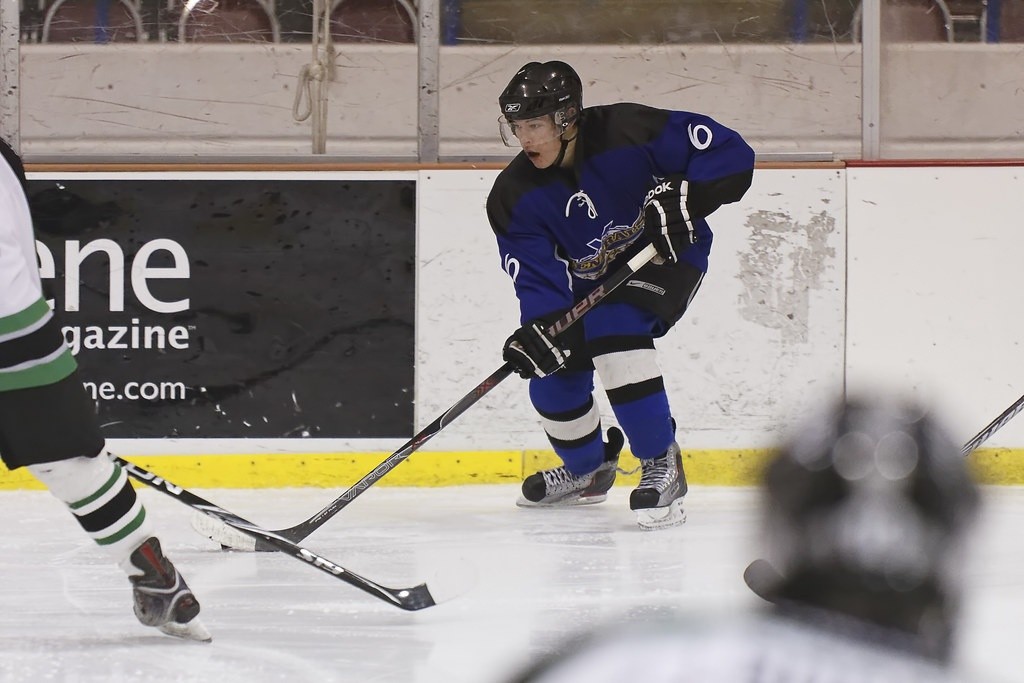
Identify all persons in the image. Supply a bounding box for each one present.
[508,391,1023,683]
[0,137,211,642]
[485,61,756,530]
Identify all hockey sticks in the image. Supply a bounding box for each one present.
[958,394,1023,458]
[100,439,456,611]
[192,232,660,552]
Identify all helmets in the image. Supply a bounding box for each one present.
[752,393,981,666]
[497,60,583,175]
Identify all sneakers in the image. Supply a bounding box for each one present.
[516,426,624,508]
[128,536,212,643]
[629,417,688,530]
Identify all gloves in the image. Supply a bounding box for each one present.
[502,320,575,380]
[644,177,715,264]
